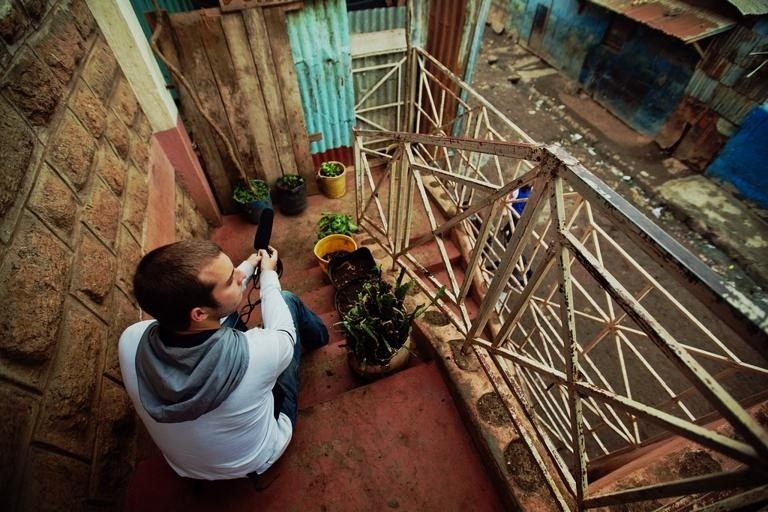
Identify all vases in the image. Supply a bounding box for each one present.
[314,233,378,318]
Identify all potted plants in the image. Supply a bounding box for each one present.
[230,159,349,226]
[331,261,449,379]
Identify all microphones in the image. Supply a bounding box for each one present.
[253,207,274,278]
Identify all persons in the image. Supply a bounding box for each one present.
[119,239,330,487]
